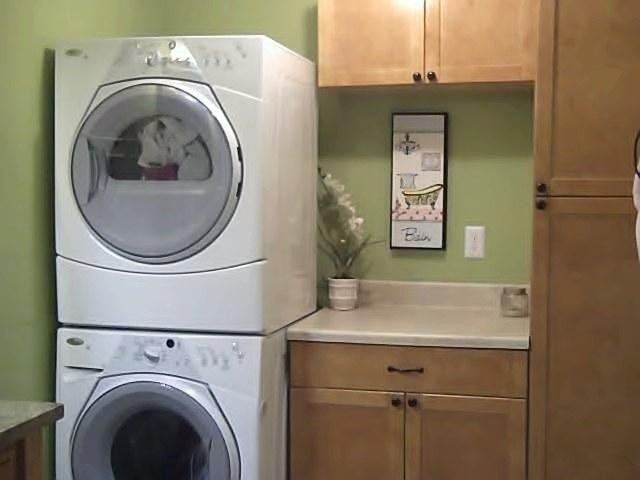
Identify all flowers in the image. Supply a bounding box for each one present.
[315,166,390,274]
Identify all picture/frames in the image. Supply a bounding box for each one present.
[390,111,448,251]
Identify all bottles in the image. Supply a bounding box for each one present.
[500,285,528,316]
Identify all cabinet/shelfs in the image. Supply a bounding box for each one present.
[315,0,534,94]
[284,340,529,480]
[529,0,637,479]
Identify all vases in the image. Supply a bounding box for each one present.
[328,277,361,312]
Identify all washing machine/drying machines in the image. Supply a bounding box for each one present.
[54,33,319,333]
[56,327,286,480]
